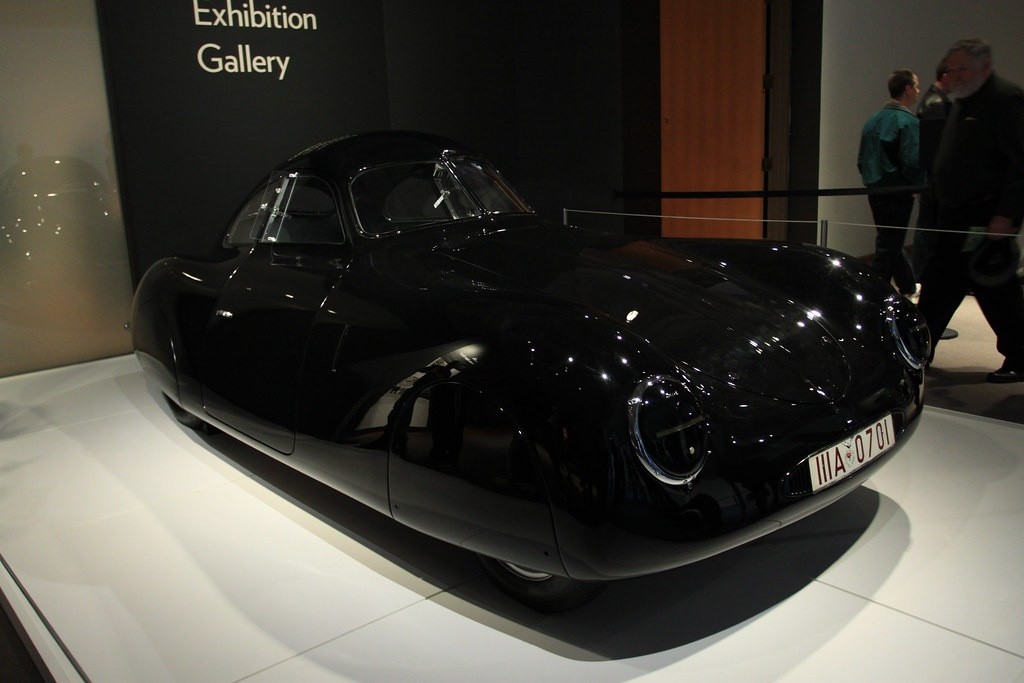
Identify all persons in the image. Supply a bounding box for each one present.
[857,67,922,306]
[912,56,954,284]
[916,36,1023,383]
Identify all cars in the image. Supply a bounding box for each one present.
[128,132,941,586]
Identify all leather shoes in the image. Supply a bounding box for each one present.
[988,367,1024,383]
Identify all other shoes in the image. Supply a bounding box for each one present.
[901,283,922,298]
